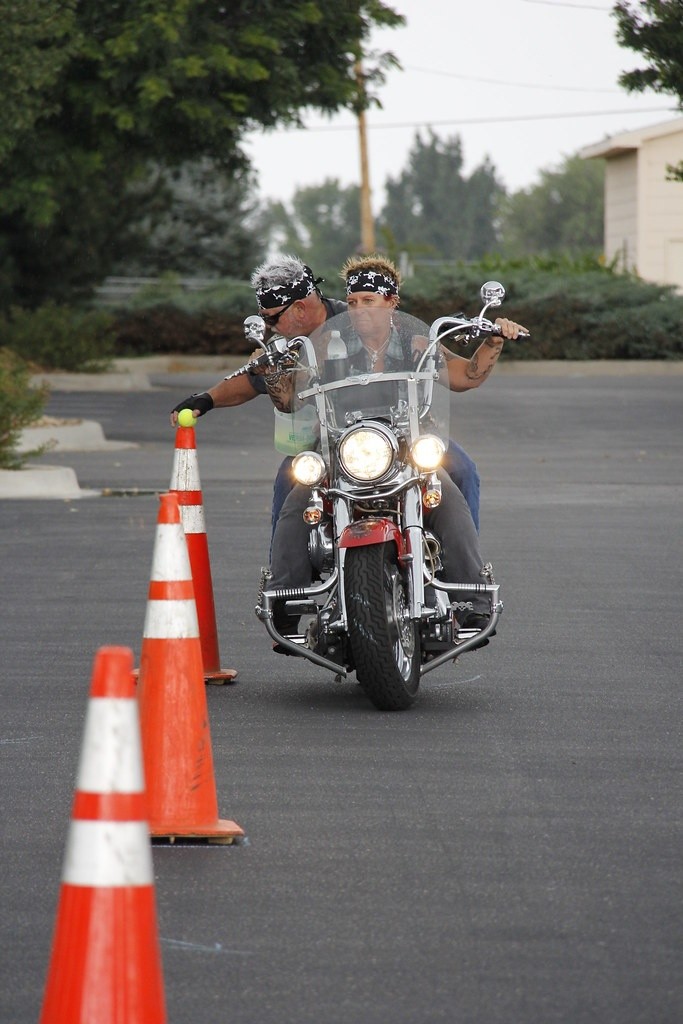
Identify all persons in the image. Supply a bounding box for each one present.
[246,256,529,656]
[170,255,490,652]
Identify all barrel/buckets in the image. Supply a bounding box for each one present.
[274,403,319,457]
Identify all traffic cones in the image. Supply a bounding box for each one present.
[36,643,169,1023]
[137,488,247,842]
[132,423,240,684]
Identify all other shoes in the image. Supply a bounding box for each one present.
[461,613,497,638]
[274,595,307,637]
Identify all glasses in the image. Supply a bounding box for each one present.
[262,299,298,326]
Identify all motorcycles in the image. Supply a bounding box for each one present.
[243,281,533,713]
